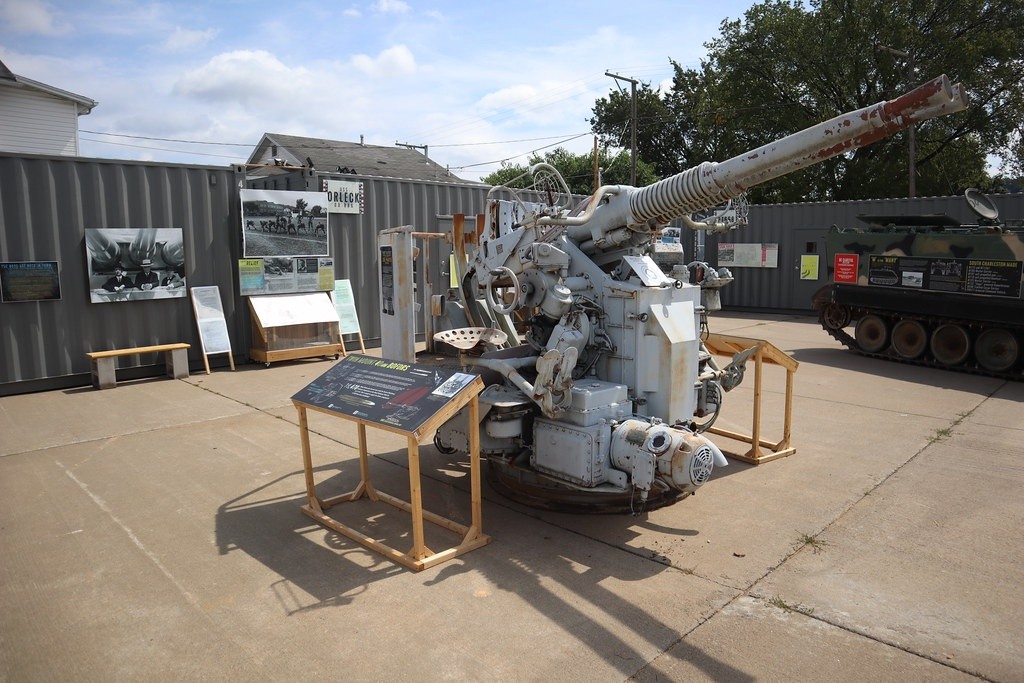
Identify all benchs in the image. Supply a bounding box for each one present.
[83,343,191,389]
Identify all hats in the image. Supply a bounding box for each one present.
[139,260,154,266]
[164,266,176,271]
[113,263,124,269]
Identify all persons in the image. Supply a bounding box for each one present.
[101,259,184,292]
[246,212,326,271]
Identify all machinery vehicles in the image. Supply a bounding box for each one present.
[811,188,1024,383]
[85,228,186,303]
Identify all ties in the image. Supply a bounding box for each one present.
[147,272,148,276]
[169,277,170,279]
[118,275,121,281]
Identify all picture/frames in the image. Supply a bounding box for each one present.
[0,261,62,303]
[239,187,330,258]
